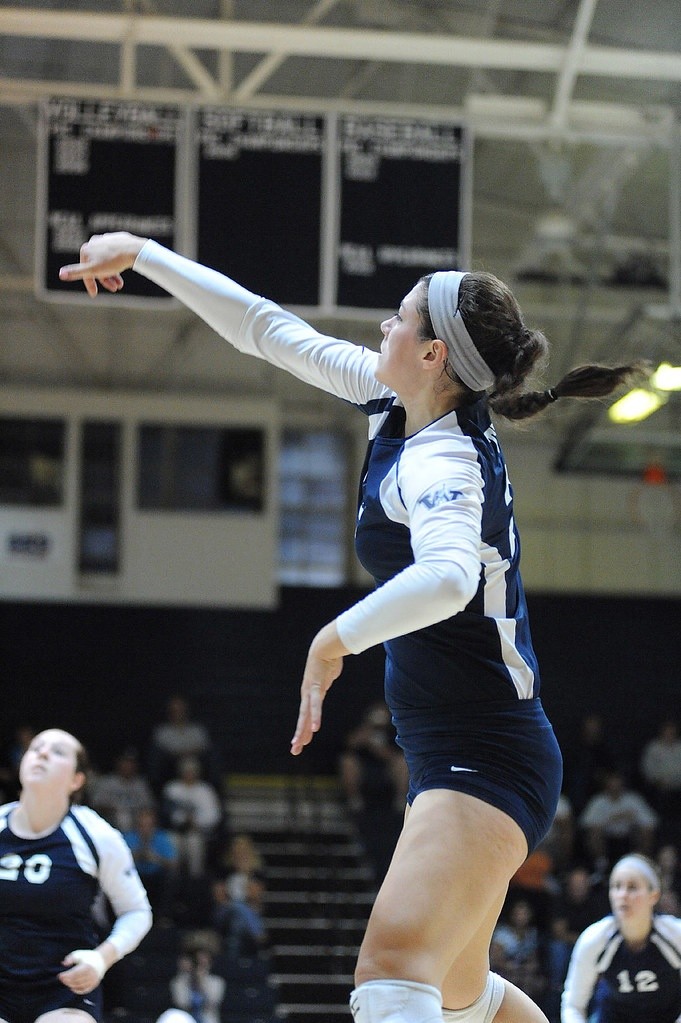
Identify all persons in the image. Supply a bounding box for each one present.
[60,231,564,1023]
[74,692,280,1022]
[344,694,680,998]
[1,728,152,1023]
[560,855,680,1023]
